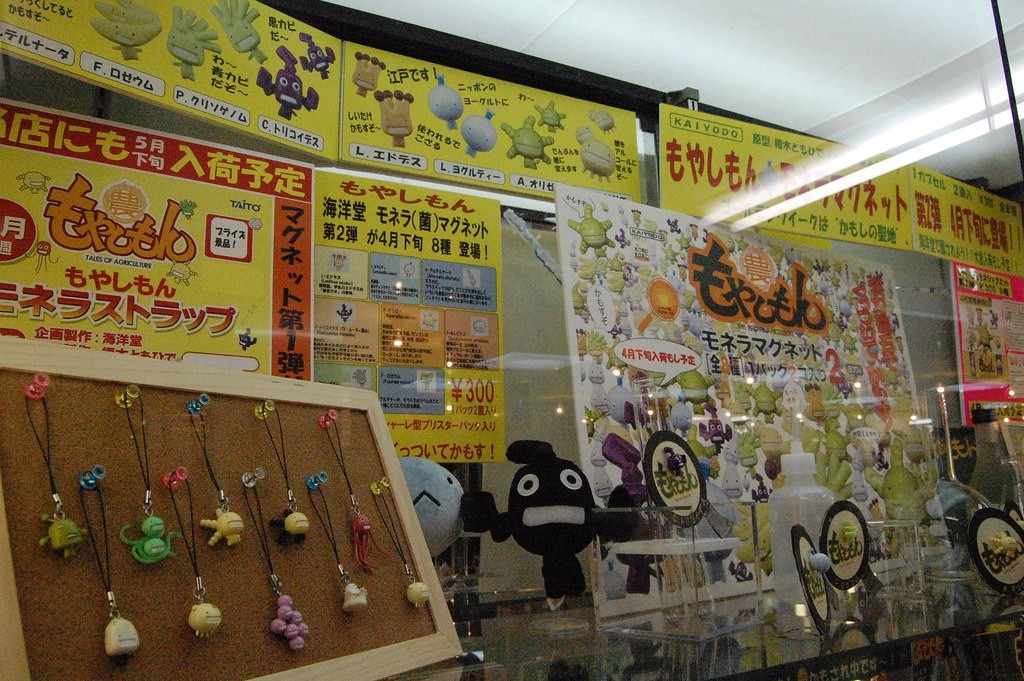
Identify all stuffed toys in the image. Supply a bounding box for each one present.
[400,457,465,558]
[461,440,636,599]
